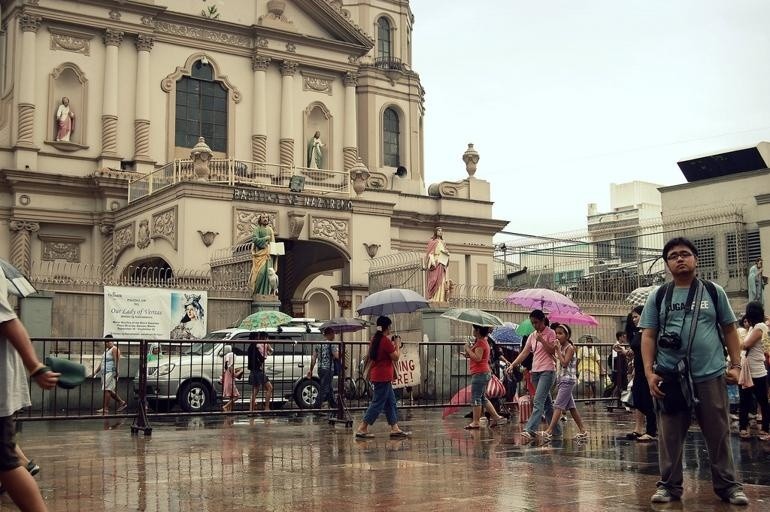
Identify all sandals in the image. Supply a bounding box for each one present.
[521,430,552,441]
[572,431,588,440]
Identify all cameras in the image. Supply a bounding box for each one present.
[658,332,682,350]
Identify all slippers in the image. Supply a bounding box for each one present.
[490,417,505,428]
[464,423,480,429]
[389,430,408,437]
[355,430,375,438]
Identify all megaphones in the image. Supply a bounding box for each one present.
[392,166,407,179]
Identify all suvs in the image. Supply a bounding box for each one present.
[132,315,338,412]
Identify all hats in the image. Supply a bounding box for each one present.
[183,293,204,311]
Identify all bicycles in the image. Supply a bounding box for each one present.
[343,365,374,401]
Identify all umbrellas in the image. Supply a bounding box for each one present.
[355,289,428,336]
[318,317,369,341]
[439,307,503,341]
[239,311,294,331]
[1,258,38,299]
[503,288,580,331]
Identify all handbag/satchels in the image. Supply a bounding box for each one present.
[46,356,85,390]
[652,356,700,414]
[483,372,509,399]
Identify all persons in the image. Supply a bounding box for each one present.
[748,257,766,309]
[577,336,605,404]
[425,227,451,302]
[0,444,41,495]
[256,331,273,371]
[248,213,277,294]
[309,131,328,168]
[171,293,206,339]
[605,330,631,412]
[723,302,770,440]
[625,305,661,439]
[509,309,587,440]
[248,332,272,418]
[223,352,244,412]
[308,328,341,417]
[56,96,75,141]
[460,323,523,429]
[90,334,128,412]
[148,343,164,362]
[636,237,749,505]
[0,266,61,512]
[356,316,408,438]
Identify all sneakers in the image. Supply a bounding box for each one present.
[116,400,127,412]
[25,459,40,476]
[739,426,770,440]
[626,430,657,441]
[726,485,749,504]
[97,407,109,413]
[551,434,564,440]
[650,485,679,502]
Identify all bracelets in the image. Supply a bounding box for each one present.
[731,362,742,369]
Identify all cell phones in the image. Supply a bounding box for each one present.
[461,352,465,355]
